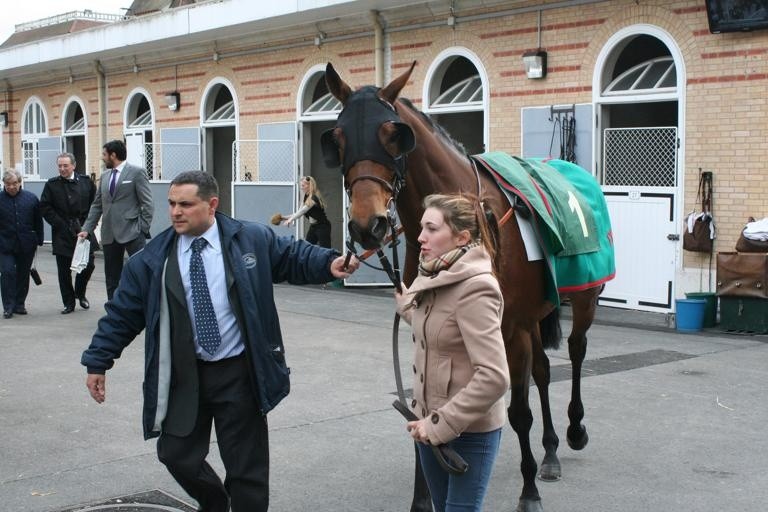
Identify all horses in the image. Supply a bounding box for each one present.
[324,60,606,512]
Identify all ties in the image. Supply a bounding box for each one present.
[109,169,118,197]
[188,238,222,356]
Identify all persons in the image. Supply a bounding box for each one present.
[39,153,100,314]
[1,168,44,319]
[78,140,155,301]
[281,175,332,248]
[408,195,511,511]
[80,170,360,512]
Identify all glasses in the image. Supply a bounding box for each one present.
[306,176,310,181]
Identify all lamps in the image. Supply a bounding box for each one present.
[521,9,548,80]
[0,91,9,128]
[163,64,181,112]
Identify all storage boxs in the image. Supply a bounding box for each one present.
[715,251,768,295]
[720,297,767,334]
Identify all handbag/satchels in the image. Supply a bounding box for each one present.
[682,171,715,254]
[30,247,42,285]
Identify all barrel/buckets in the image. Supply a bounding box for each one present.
[685,292,717,328]
[674,298,706,331]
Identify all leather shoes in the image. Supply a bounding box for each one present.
[14,306,27,314]
[75,293,90,309]
[4,306,14,318]
[60,304,75,314]
